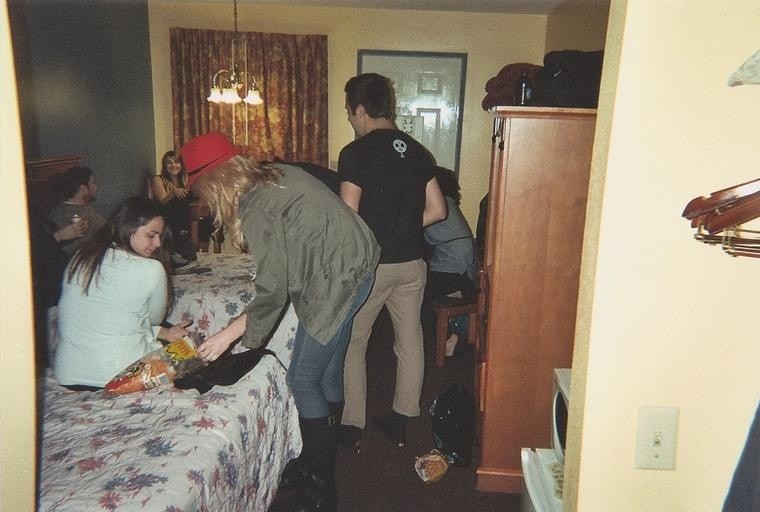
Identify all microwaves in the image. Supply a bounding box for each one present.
[550,368,573,460]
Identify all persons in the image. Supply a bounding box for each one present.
[29,214,90,368]
[198,213,225,253]
[179,132,383,509]
[423,167,477,356]
[49,167,108,258]
[151,151,198,260]
[337,72,449,449]
[52,193,193,391]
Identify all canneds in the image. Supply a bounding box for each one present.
[71,213,81,222]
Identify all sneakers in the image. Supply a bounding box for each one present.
[336,432,360,460]
[377,416,405,448]
[445,334,458,356]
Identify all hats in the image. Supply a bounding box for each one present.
[180,131,243,185]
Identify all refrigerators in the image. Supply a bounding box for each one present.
[518,446,564,512]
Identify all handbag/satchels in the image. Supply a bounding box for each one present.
[429,383,474,466]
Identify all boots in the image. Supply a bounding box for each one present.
[268,402,344,512]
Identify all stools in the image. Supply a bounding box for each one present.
[424,296,480,369]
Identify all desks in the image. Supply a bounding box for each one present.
[513,444,562,512]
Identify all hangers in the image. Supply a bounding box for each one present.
[681,179,760,258]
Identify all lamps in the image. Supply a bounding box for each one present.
[207,1,267,106]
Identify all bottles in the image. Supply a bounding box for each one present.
[71,214,83,223]
[515,68,533,106]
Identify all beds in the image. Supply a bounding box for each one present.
[35,250,303,512]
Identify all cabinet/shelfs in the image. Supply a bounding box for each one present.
[473,104,600,495]
